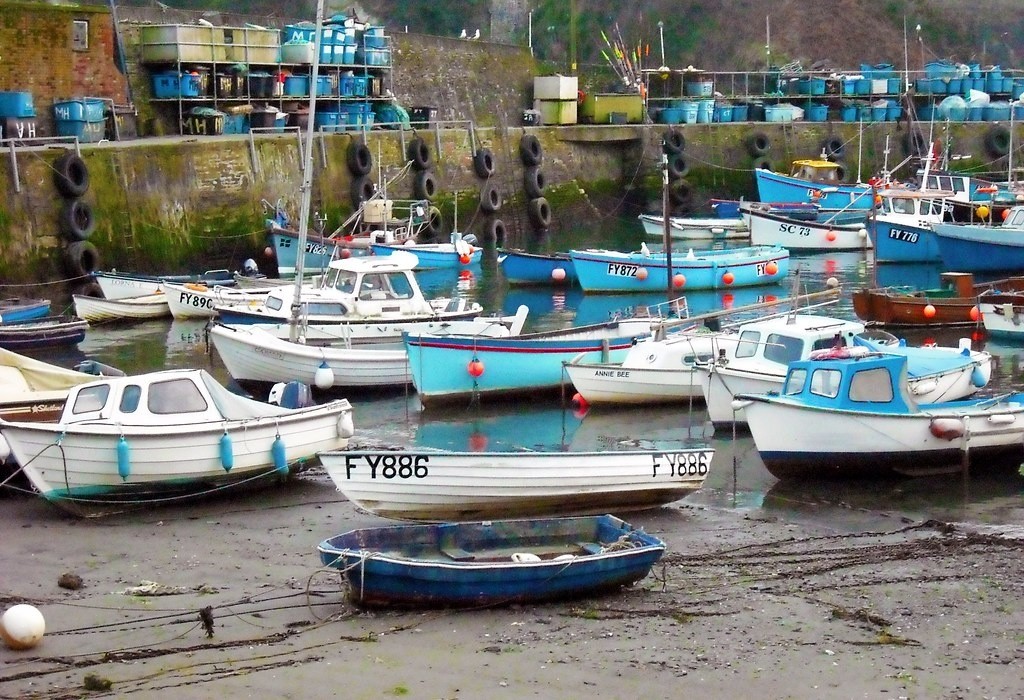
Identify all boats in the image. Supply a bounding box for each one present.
[401,294,705,411]
[212,250,483,325]
[0,348,128,468]
[496,247,579,288]
[637,96,1024,265]
[732,345,1023,486]
[371,189,483,271]
[930,206,1024,275]
[317,513,667,612]
[0,296,89,349]
[679,262,991,432]
[270,141,430,279]
[0,368,355,518]
[72,268,314,323]
[977,288,1024,342]
[318,448,717,523]
[851,272,1024,329]
[568,246,791,295]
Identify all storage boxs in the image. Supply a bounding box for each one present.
[363,198,395,223]
[531,62,1024,127]
[0,19,398,142]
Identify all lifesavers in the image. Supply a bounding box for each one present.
[745,131,770,158]
[520,135,552,241]
[474,148,507,240]
[664,153,689,178]
[988,125,1012,181]
[408,139,443,238]
[184,283,208,292]
[751,156,776,181]
[668,179,695,218]
[903,129,929,156]
[662,130,685,155]
[832,161,850,184]
[821,136,846,160]
[64,240,101,282]
[59,199,95,240]
[810,346,869,359]
[977,187,997,193]
[53,155,90,197]
[347,141,376,209]
[69,283,105,316]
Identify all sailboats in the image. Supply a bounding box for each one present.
[208,0,530,401]
[562,286,843,409]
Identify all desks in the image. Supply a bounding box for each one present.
[941,272,973,297]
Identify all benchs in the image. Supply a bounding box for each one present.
[577,542,603,555]
[441,546,475,562]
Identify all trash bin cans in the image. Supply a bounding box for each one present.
[523,113,540,125]
[612,112,627,124]
[410,106,438,130]
[251,112,276,134]
